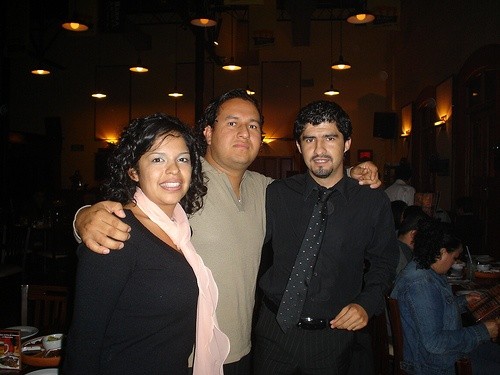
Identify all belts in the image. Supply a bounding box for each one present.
[266,298,336,331]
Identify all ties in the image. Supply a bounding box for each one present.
[276,187,338,334]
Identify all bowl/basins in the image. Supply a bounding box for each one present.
[42,333,64,351]
[477,264,490,273]
[451,263,464,270]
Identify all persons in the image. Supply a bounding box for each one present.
[252,98,402,375]
[57,111,231,375]
[72,88,382,375]
[390,226,500,375]
[383,166,499,375]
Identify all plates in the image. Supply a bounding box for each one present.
[20,336,61,366]
[5,326,39,339]
[0,355,19,369]
[0,342,9,354]
[24,368,58,375]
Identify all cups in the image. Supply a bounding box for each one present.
[466,257,477,272]
[49,208,64,225]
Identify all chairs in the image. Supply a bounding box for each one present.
[367,292,472,375]
[25,284,74,337]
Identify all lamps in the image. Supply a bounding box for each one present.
[60,0,376,96]
